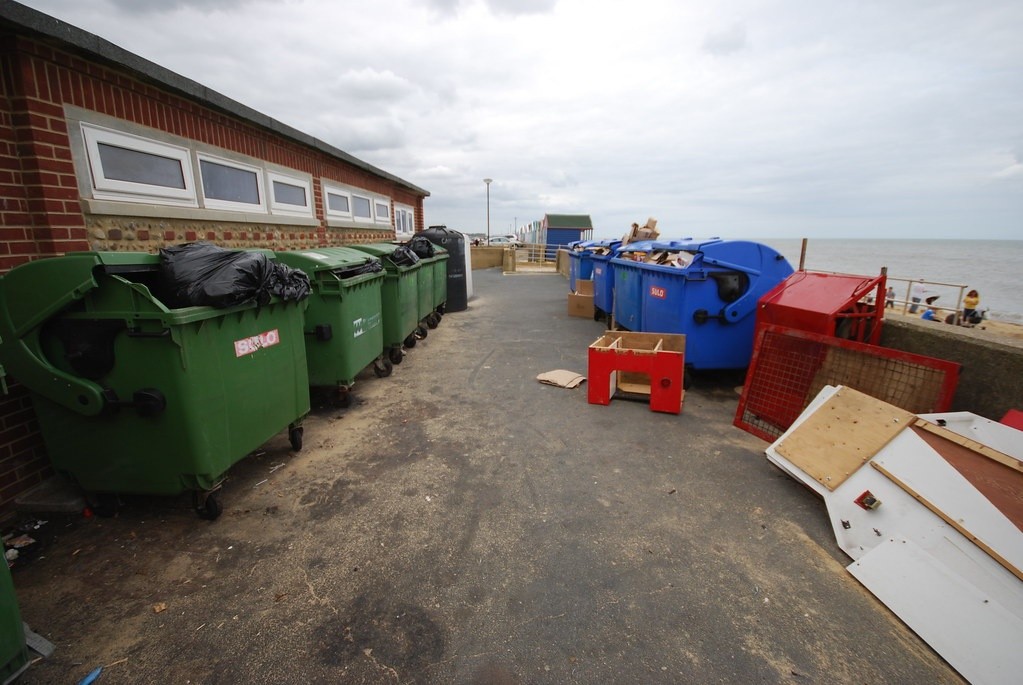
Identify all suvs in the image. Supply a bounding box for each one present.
[501,234,518,241]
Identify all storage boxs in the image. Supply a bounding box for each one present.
[566,279,595,318]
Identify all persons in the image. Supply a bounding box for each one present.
[920,307,946,322]
[908,278,928,314]
[945,311,963,326]
[962,289,980,322]
[883,286,897,310]
[473,238,479,246]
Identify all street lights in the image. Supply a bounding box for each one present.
[483,178,493,246]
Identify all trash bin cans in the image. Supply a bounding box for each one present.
[567,238,596,294]
[349,244,427,365]
[0,247,312,521]
[640,236,796,383]
[589,239,622,329]
[609,239,659,332]
[398,240,450,328]
[274,248,393,406]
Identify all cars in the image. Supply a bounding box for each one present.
[481,237,523,249]
